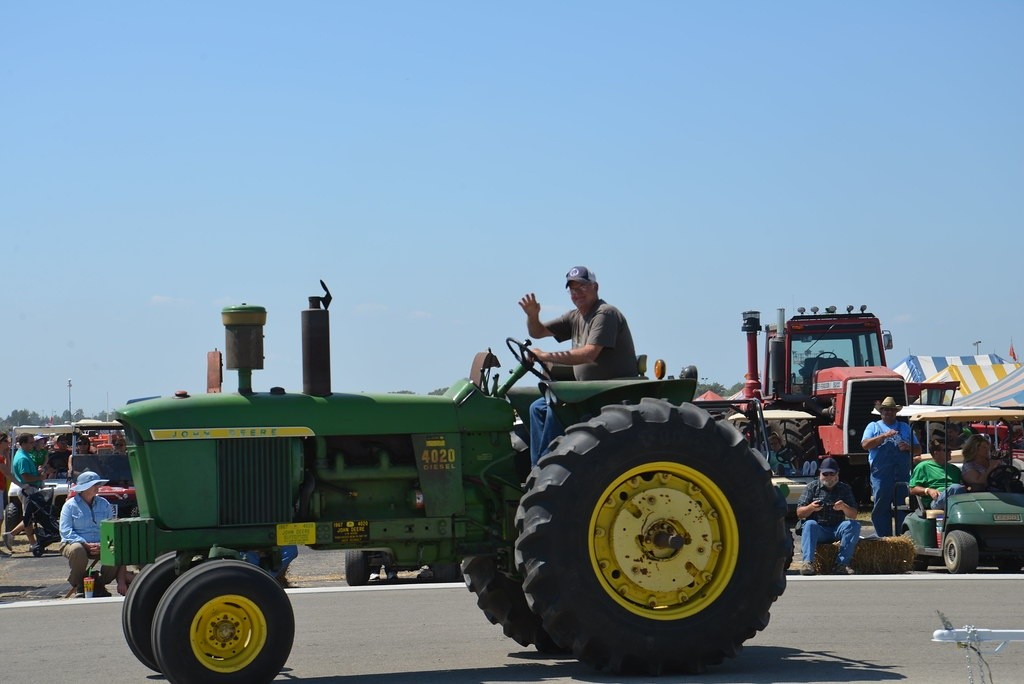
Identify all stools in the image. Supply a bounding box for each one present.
[62,555,101,598]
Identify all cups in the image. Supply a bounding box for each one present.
[84,578,94,598]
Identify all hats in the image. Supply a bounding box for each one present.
[875,397,902,411]
[76,435,90,444]
[565,265,596,288]
[57,435,68,442]
[33,435,44,441]
[819,457,839,473]
[69,471,109,491]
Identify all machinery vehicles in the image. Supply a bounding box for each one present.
[741,304,960,508]
[100,279,796,683]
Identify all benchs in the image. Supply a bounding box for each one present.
[907,483,943,519]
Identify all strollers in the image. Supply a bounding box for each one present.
[22,487,62,558]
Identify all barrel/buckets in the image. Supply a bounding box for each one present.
[936,514,944,548]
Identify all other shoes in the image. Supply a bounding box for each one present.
[73,588,85,598]
[802,461,810,477]
[809,461,818,476]
[94,587,112,597]
[0,549,12,557]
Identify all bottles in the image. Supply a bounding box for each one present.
[894,433,902,445]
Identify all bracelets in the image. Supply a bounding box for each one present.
[925,488,929,495]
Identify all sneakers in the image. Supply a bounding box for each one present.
[831,561,854,575]
[28,542,48,552]
[800,561,818,575]
[1,533,17,551]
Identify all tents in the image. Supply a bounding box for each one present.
[892,354,1024,408]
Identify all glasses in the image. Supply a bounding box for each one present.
[939,448,951,453]
[821,471,838,477]
[566,285,590,293]
[78,442,88,446]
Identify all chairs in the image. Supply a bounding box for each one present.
[804,357,844,395]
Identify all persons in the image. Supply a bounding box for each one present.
[67,436,94,469]
[0,432,29,526]
[48,435,70,479]
[797,458,860,575]
[861,396,922,537]
[948,423,966,450]
[29,435,49,469]
[518,266,639,467]
[961,434,1001,492]
[59,471,139,598]
[909,439,965,510]
[369,565,398,580]
[2,432,49,553]
